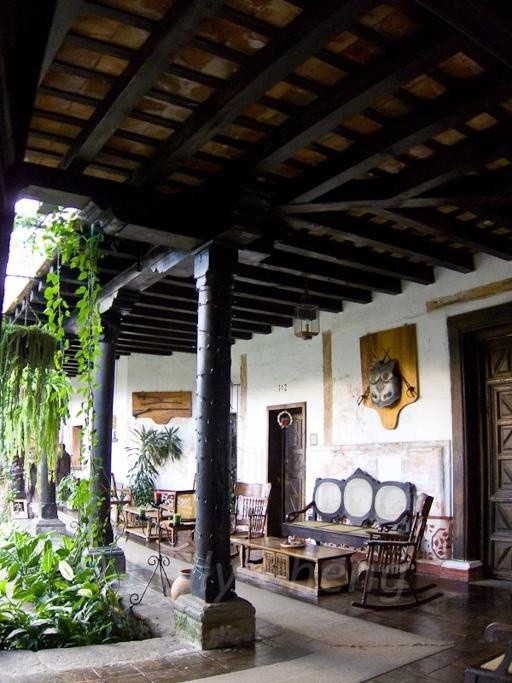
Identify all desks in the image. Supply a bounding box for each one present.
[231,537,356,597]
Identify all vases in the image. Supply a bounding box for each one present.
[171,568,195,604]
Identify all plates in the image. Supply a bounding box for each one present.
[279,539,307,548]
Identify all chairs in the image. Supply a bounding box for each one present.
[352,494,445,611]
[188,482,272,565]
[110,473,196,553]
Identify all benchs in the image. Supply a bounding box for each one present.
[281,468,414,553]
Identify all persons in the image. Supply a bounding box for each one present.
[56,444,70,485]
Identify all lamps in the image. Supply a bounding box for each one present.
[292,271,321,339]
